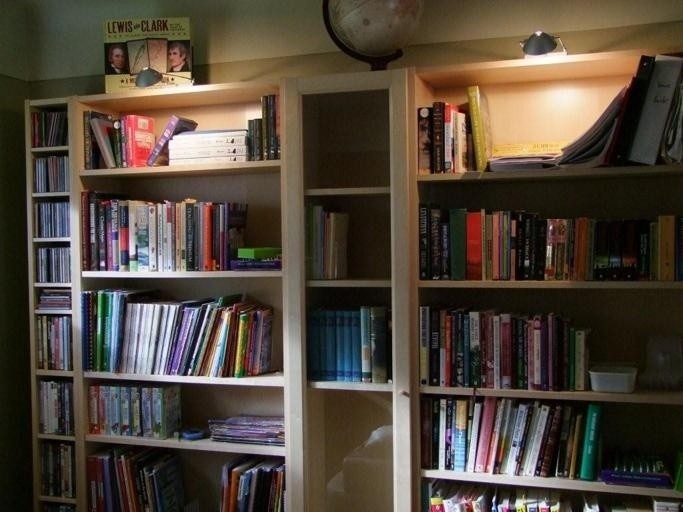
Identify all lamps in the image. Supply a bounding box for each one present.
[134,66,194,87]
[520,29,568,61]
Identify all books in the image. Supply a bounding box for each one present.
[80,287,274,378]
[82,94,279,170]
[309,203,350,279]
[87,384,286,447]
[419,301,592,388]
[417,53,683,168]
[33,103,76,512]
[307,300,392,383]
[81,188,283,273]
[419,194,681,282]
[86,445,287,512]
[421,396,682,512]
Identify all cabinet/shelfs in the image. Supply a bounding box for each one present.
[71,81,289,512]
[282,68,412,512]
[412,51,682,512]
[24,96,82,511]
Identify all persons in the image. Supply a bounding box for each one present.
[168,41,190,71]
[105,44,128,74]
